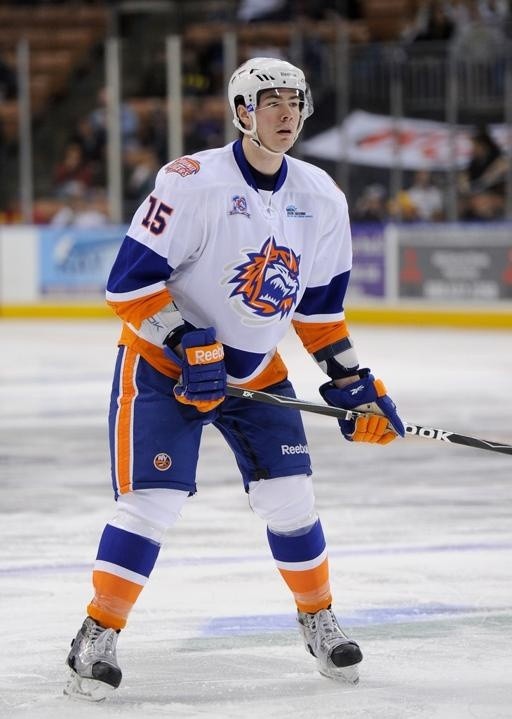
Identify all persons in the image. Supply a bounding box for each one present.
[64,54,406,688]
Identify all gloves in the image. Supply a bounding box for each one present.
[318,367,405,446]
[163,327,228,426]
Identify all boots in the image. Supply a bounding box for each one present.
[296,604,363,668]
[65,616,122,690]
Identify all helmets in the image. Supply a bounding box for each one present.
[227,57,314,135]
